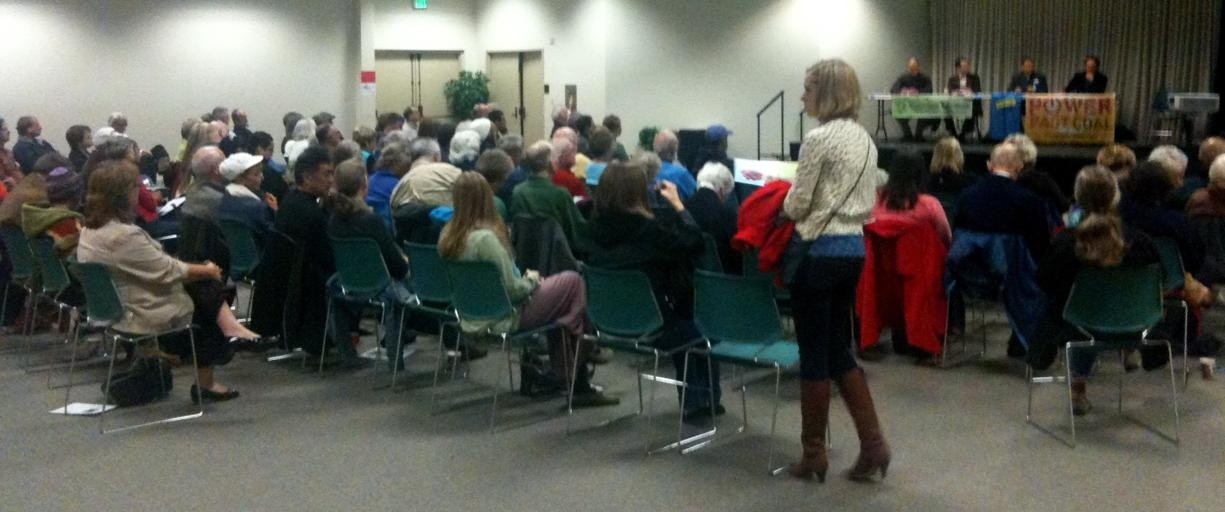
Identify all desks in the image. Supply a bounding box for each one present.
[867,93,1026,143]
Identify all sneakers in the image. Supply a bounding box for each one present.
[572,389,619,407]
[580,344,612,363]
[450,345,486,364]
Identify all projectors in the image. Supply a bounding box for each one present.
[1167,91,1220,114]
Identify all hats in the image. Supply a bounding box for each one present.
[705,125,730,142]
[219,152,263,180]
[47,167,82,202]
[92,127,128,145]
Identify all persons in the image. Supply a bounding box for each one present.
[0,56,1225,482]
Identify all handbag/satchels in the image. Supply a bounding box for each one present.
[519,341,564,397]
[780,234,810,285]
[102,358,173,406]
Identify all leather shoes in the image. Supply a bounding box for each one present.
[224,334,278,352]
[191,385,239,404]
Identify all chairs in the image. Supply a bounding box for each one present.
[945,232,1032,373]
[391,241,514,402]
[1,218,121,388]
[678,269,832,474]
[316,242,402,389]
[567,266,718,456]
[1029,266,1182,448]
[218,216,282,354]
[65,263,204,438]
[439,258,569,431]
[856,207,941,365]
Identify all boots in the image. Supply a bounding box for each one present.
[1071,382,1091,414]
[835,366,890,479]
[787,378,831,481]
